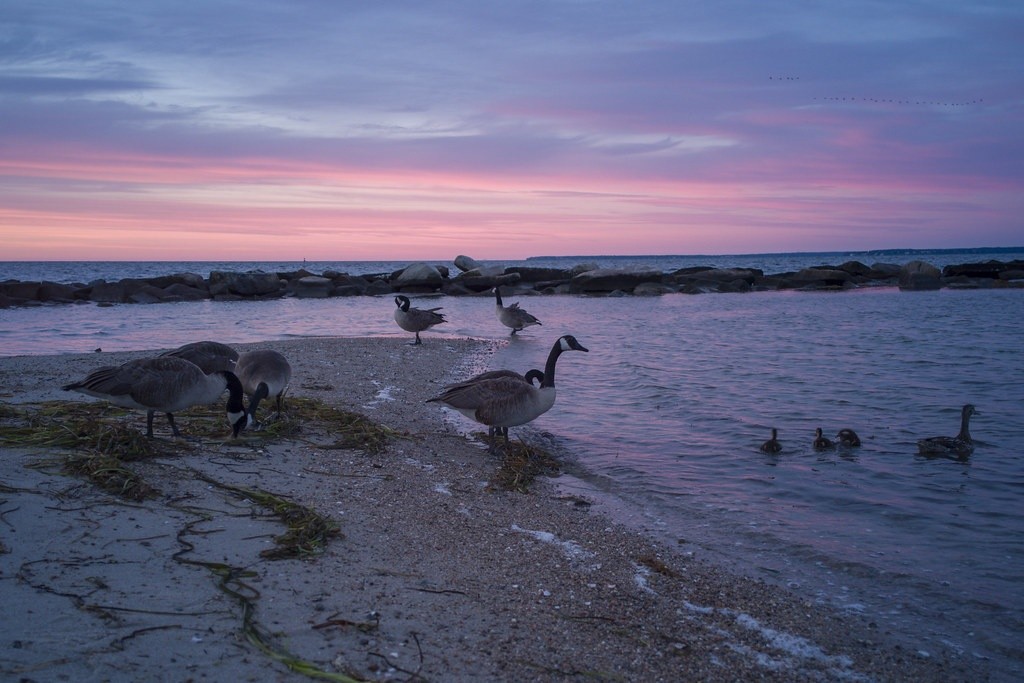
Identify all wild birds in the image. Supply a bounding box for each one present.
[60,341,292,449]
[393,295,448,347]
[759,427,783,454]
[812,426,861,450]
[915,404,982,458]
[487,287,542,337]
[424,334,591,453]
